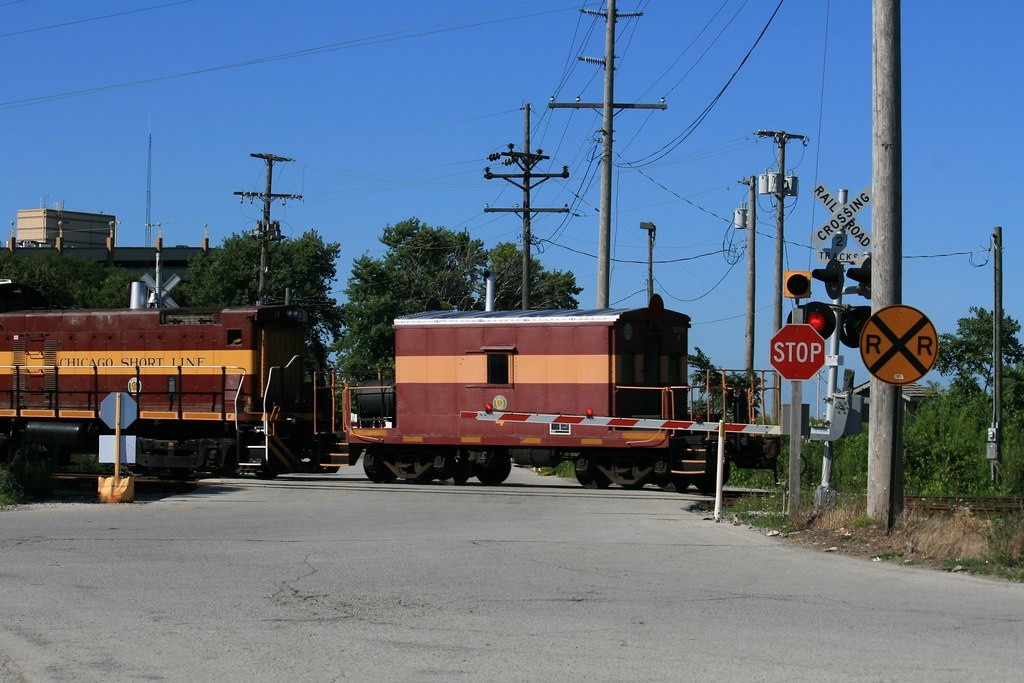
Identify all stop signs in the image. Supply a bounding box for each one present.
[770,324,825,380]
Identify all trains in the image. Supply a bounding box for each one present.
[0,294,780,507]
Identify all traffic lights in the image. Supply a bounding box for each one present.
[801,301,836,340]
[812,258,843,299]
[784,270,810,298]
[845,257,871,300]
